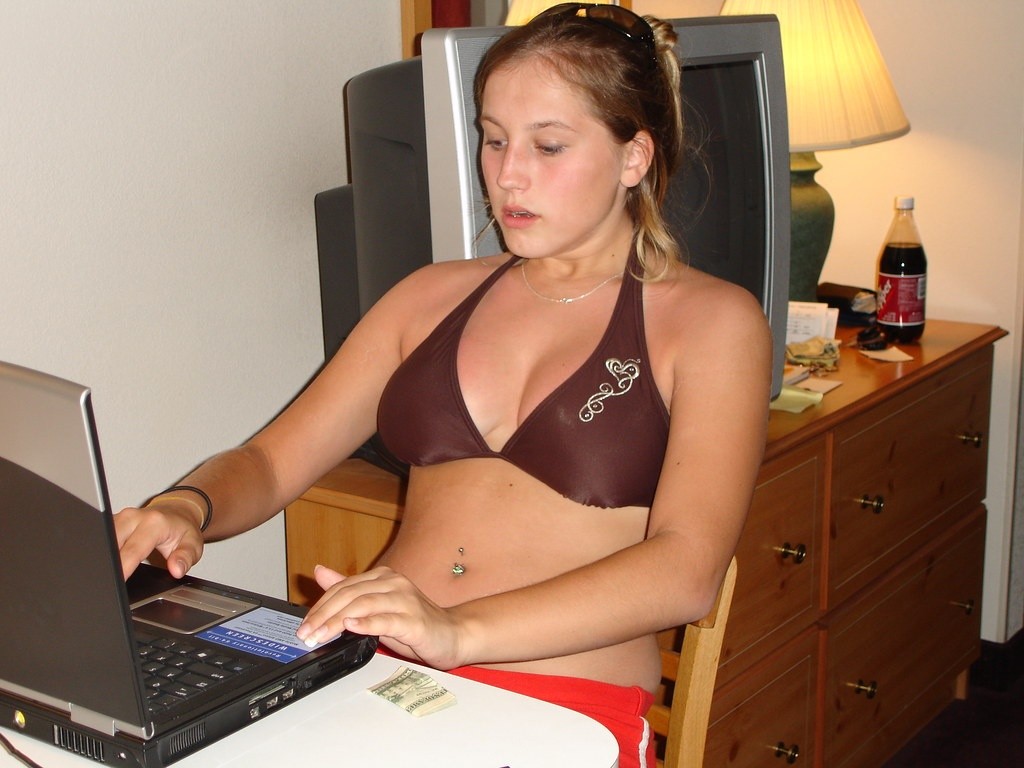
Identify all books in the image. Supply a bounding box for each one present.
[783,364,810,386]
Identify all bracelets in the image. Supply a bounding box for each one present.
[147,487,213,531]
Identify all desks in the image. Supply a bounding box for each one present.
[0,653,620,768]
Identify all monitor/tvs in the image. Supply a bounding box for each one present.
[314,13,792,476]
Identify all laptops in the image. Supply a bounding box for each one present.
[0,362,380,768]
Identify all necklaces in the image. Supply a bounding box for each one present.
[522,257,626,303]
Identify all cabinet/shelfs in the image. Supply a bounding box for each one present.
[286,320,1010,767]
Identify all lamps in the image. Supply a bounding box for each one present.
[720,0,912,302]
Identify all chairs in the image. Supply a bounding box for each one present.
[646,556,737,768]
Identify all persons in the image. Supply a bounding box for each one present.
[112,3,773,768]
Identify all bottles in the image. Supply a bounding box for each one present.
[875,196,927,342]
